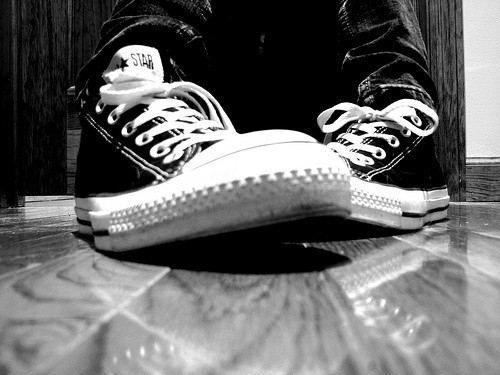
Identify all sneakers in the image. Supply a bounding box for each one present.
[72,42,352,250]
[326,96,449,231]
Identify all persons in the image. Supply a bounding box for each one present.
[73,0,451,253]
